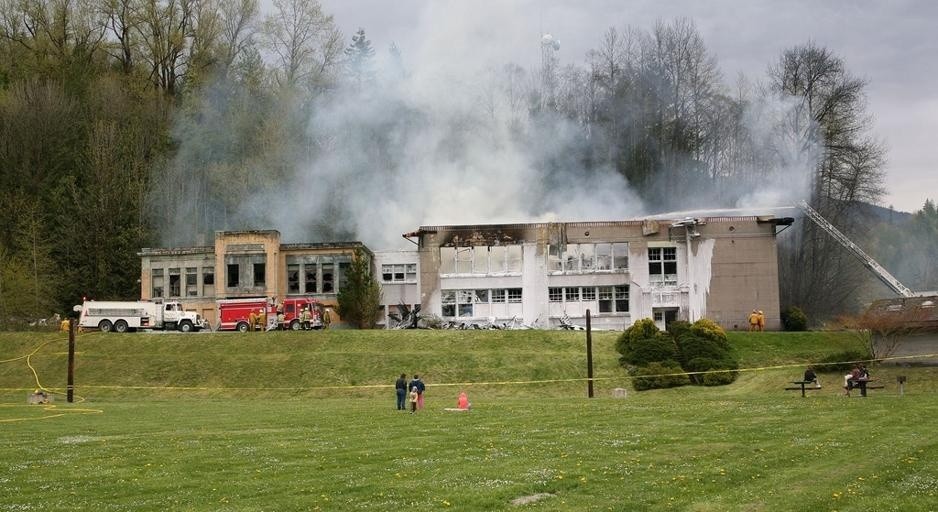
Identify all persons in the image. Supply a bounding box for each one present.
[758,311,764,332]
[324,308,331,329]
[258,309,266,332]
[805,364,822,388]
[276,303,284,314]
[277,314,288,331]
[299,308,304,329]
[249,308,258,332]
[395,372,425,415]
[60,317,70,334]
[846,365,868,397]
[457,392,470,409]
[302,307,311,330]
[749,310,758,331]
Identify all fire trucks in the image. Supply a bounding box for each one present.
[72,297,210,332]
[213,297,326,333]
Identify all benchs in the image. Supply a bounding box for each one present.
[844,379,884,397]
[786,381,821,397]
